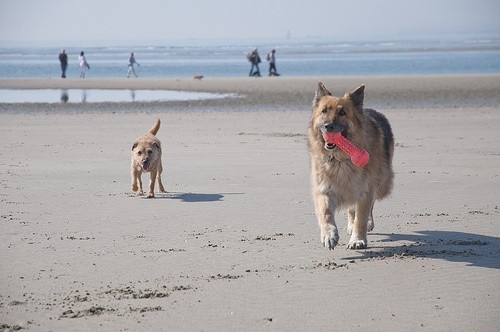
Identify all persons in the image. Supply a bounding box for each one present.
[269,49,278,75]
[79,50,91,77]
[126,52,139,77]
[248,48,261,77]
[59,49,69,77]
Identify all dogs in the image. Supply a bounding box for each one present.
[192,72,204,80]
[130,116,168,199]
[307,80,395,251]
